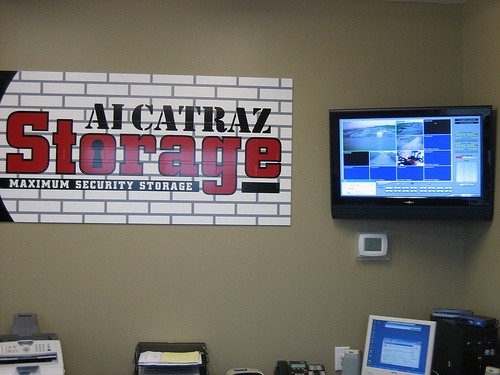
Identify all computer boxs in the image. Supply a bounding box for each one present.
[431,313,499,375]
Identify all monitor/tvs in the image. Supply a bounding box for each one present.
[329,105,494,219]
[360,314,436,375]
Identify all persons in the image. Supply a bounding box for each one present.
[398,152,423,166]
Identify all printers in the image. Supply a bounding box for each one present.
[0,312,65,375]
[135,342,209,375]
